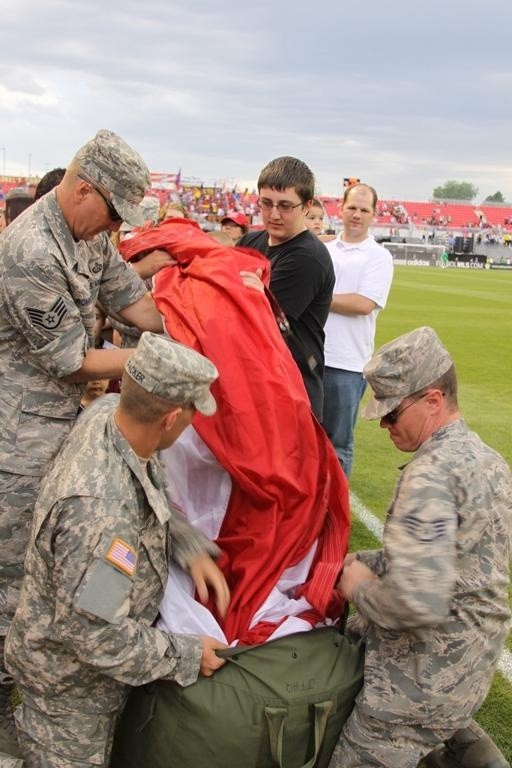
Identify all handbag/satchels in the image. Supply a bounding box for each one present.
[111,628,362,768]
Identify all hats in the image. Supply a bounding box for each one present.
[125,331,218,416]
[221,212,249,226]
[361,326,453,419]
[76,129,160,227]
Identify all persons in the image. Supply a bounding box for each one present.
[6,332,221,767]
[395,199,512,266]
[164,155,393,486]
[2,167,66,218]
[1,129,152,685]
[332,328,512,767]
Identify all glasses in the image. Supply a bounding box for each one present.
[382,392,446,424]
[77,175,122,221]
[256,197,311,213]
